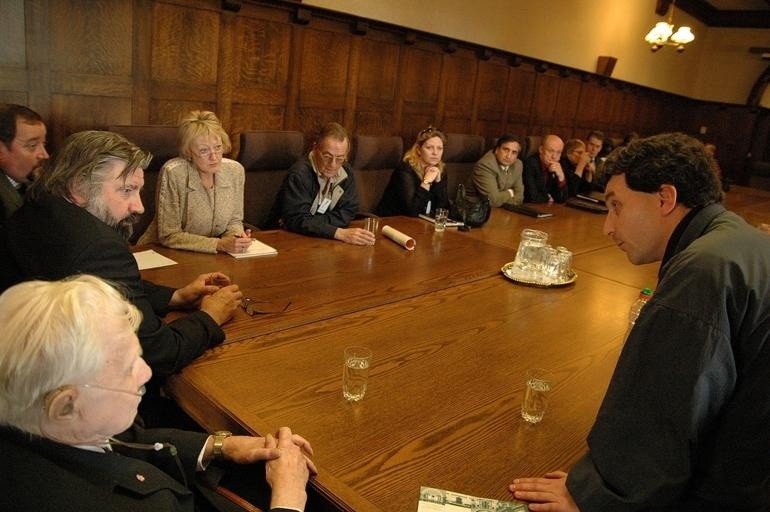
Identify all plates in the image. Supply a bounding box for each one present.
[501,261,578,286]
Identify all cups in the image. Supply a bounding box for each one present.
[520,368,554,423]
[544,245,572,282]
[512,227,549,277]
[365,218,378,234]
[342,347,371,401]
[435,208,448,232]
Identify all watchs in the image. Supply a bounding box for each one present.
[211,430,233,459]
[423,178,432,185]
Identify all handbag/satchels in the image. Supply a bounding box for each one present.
[449,184,491,227]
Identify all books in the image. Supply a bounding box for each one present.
[227,238,279,260]
[565,193,609,214]
[502,203,553,219]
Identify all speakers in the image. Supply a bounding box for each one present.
[598,55,617,77]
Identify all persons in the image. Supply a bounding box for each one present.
[465,135,525,208]
[1,273,318,512]
[137,110,255,254]
[2,103,245,432]
[374,125,450,218]
[256,122,376,247]
[522,130,727,204]
[508,131,770,512]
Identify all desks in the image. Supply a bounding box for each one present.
[128,184,770,512]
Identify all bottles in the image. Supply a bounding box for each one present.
[621,287,652,342]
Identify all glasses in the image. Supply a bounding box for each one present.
[241,296,292,318]
[316,144,348,166]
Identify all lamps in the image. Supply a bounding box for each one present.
[644,0,695,54]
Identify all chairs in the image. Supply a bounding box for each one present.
[235,130,303,230]
[523,136,543,160]
[103,125,178,246]
[350,132,403,217]
[442,133,485,200]
